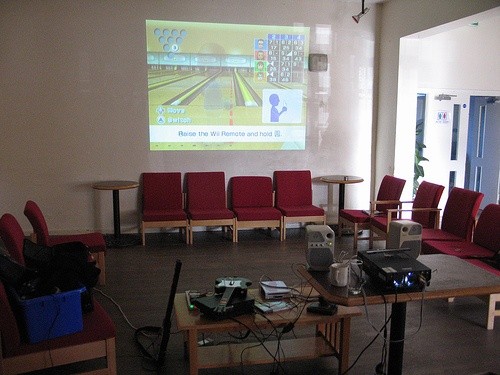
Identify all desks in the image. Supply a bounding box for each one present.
[320,175,364,236]
[88,181,140,247]
[298,254,500,375]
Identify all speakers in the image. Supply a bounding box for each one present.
[304,224,336,272]
[389,219,423,258]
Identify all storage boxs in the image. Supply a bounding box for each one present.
[6,273,87,343]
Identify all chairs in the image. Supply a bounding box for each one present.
[0,201,117,375]
[230,176,283,242]
[140,173,188,244]
[275,171,326,240]
[339,175,500,330]
[186,172,235,244]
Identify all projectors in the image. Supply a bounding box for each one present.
[357,248,432,289]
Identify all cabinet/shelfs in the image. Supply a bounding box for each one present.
[174,286,362,374]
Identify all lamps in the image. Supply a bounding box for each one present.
[352,0,369,24]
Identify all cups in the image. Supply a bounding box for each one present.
[330,263,349,287]
